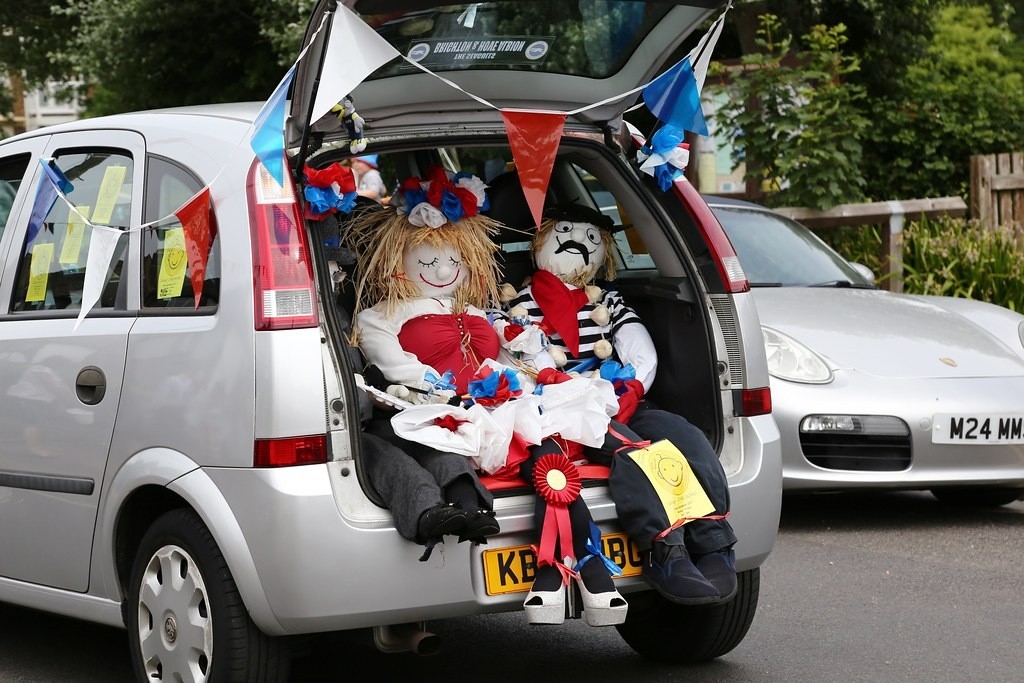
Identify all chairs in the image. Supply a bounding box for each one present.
[485,171,559,288]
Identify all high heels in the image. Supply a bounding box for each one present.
[573,558,628,626]
[523,560,573,625]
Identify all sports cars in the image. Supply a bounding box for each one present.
[583,191,1020,506]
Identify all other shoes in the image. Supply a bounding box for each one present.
[695,546,738,602]
[461,509,500,536]
[417,502,467,543]
[640,552,722,603]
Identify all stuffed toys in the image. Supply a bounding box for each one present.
[297,158,502,550]
[343,166,631,626]
[505,202,741,606]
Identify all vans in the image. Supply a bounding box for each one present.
[0,0,782,683]
